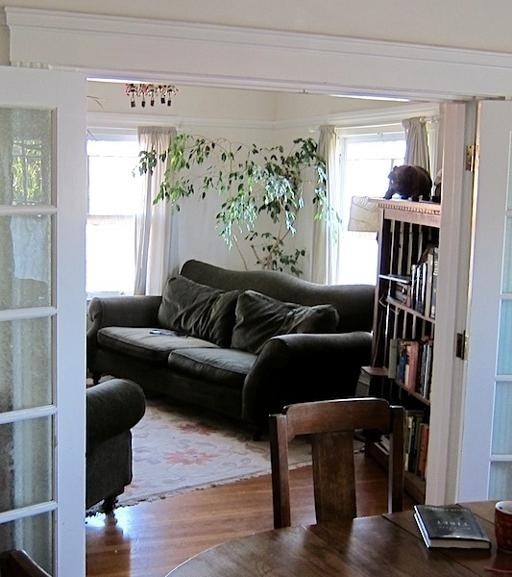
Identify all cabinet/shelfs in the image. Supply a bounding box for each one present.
[365,197,441,505]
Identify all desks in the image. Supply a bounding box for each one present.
[165,499,511,577]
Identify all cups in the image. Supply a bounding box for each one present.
[494,500,512,555]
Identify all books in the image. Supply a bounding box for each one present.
[374,247,438,479]
[413,503,493,553]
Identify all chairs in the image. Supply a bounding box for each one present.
[269,397,404,529]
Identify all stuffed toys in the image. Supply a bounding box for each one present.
[383,165,432,202]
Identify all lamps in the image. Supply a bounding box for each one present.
[125,83,178,109]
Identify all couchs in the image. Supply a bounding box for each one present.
[86,380,146,512]
[86,259,377,438]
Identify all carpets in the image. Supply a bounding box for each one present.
[87,403,364,516]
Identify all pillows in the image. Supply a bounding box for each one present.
[156,276,240,348]
[231,291,339,354]
